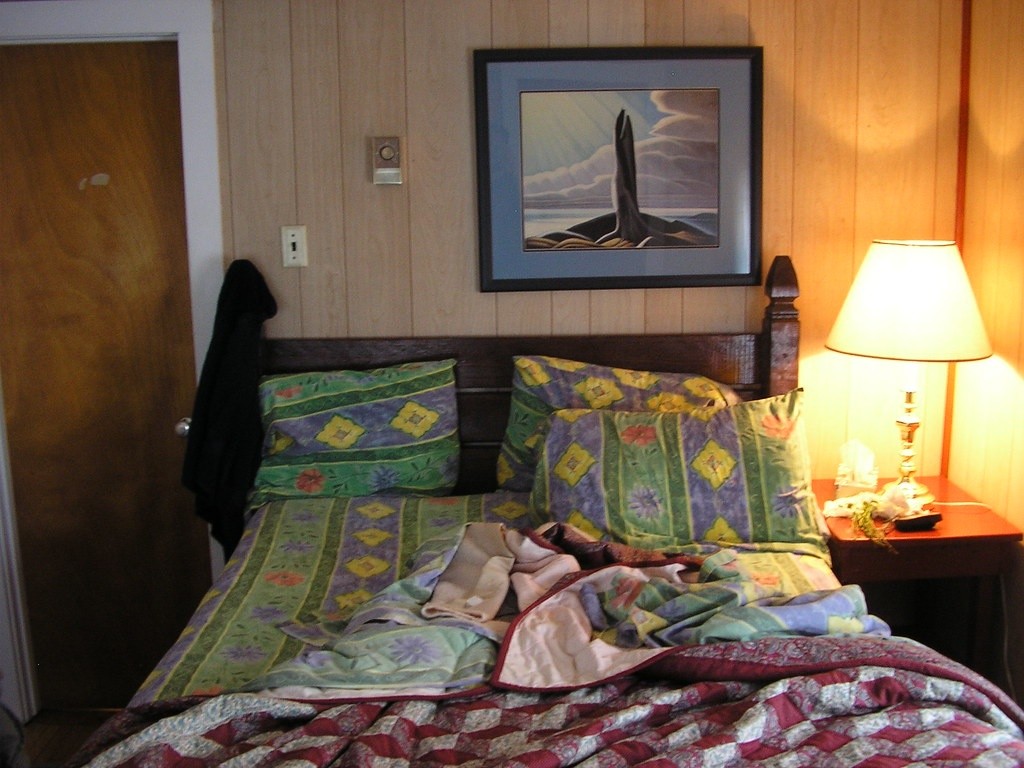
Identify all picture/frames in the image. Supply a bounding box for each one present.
[471,46,764,294]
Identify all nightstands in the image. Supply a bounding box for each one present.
[805,476,1023,687]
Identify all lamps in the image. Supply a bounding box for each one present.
[825,239,994,513]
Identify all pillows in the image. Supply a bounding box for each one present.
[497,357,741,492]
[239,359,458,520]
[529,388,835,570]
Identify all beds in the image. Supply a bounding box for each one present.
[58,258,1024,768]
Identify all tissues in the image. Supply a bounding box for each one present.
[834,440,879,499]
